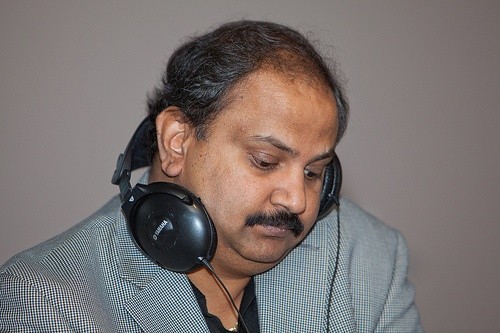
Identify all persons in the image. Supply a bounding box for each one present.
[1,19,422,332]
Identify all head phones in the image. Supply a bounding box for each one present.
[110,114,343,274]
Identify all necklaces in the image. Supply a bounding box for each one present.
[227,322,238,332]
